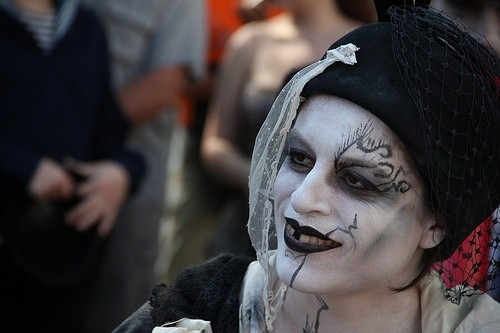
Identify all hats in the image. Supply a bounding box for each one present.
[281,5,500,262]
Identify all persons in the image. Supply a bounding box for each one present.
[113,0,500,333]
[87,0,209,323]
[201,0,366,260]
[0,0,149,333]
[431,0,500,303]
